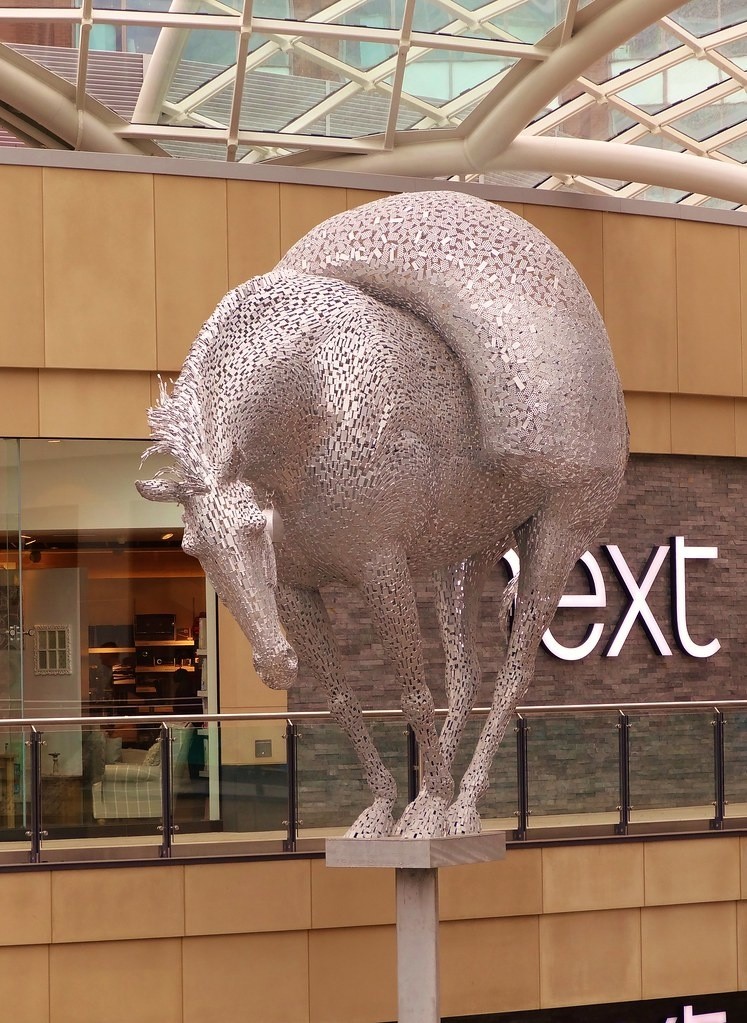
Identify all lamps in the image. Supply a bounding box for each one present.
[25,534,36,546]
[160,530,174,540]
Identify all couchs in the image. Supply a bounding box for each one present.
[91,719,194,827]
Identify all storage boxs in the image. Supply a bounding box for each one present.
[88,614,192,667]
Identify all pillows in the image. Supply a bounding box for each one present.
[104,736,123,765]
[79,732,108,785]
[142,742,161,767]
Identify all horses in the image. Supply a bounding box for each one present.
[131,185,632,855]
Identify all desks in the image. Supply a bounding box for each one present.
[0,752,20,829]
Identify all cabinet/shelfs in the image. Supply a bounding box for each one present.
[197,648,209,777]
[87,640,200,713]
